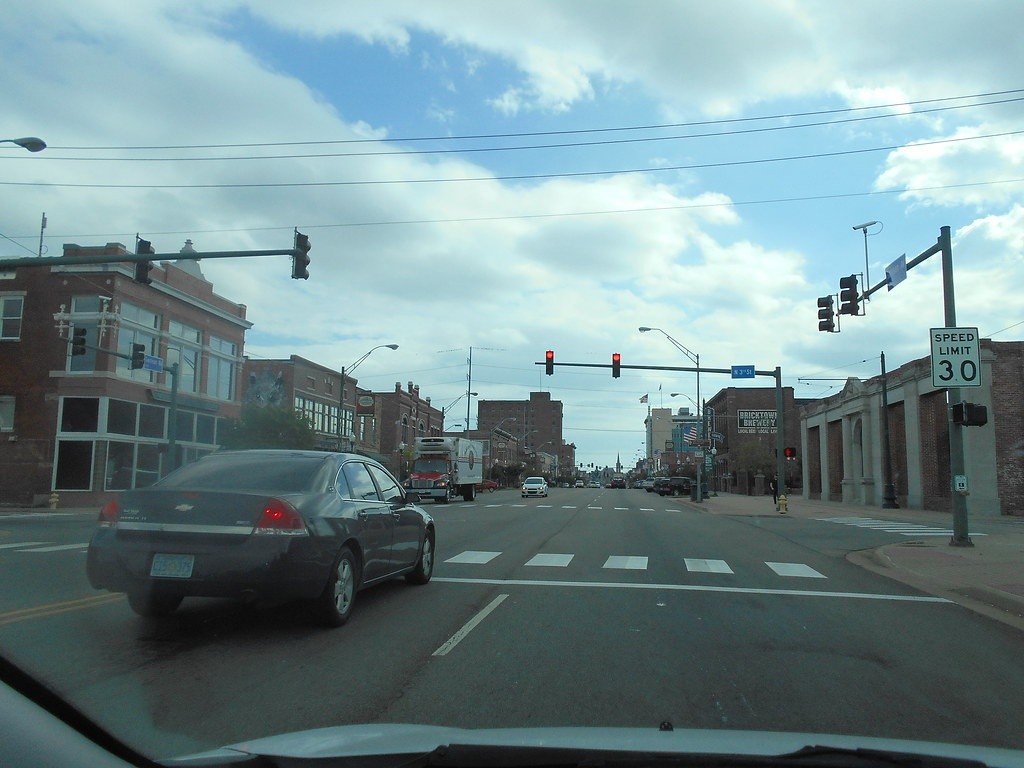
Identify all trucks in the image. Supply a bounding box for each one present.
[402,436,483,505]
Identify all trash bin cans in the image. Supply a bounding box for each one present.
[690,484,697,500]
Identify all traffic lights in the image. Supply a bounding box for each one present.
[546,350,554,375]
[818,295,835,333]
[952,398,988,429]
[131,341,145,372]
[292,231,311,282]
[613,353,621,378]
[71,326,89,356]
[840,273,860,317]
[785,446,796,459]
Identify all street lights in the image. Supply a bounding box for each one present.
[488,417,517,479]
[441,391,480,437]
[516,429,540,469]
[671,393,709,498]
[535,441,551,471]
[639,326,702,504]
[336,344,399,450]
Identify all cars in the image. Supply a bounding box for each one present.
[82,449,437,632]
[475,478,498,493]
[573,475,695,495]
[520,476,548,498]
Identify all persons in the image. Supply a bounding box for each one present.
[432,459,444,472]
[770,474,779,504]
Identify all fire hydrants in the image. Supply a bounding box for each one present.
[777,494,788,513]
